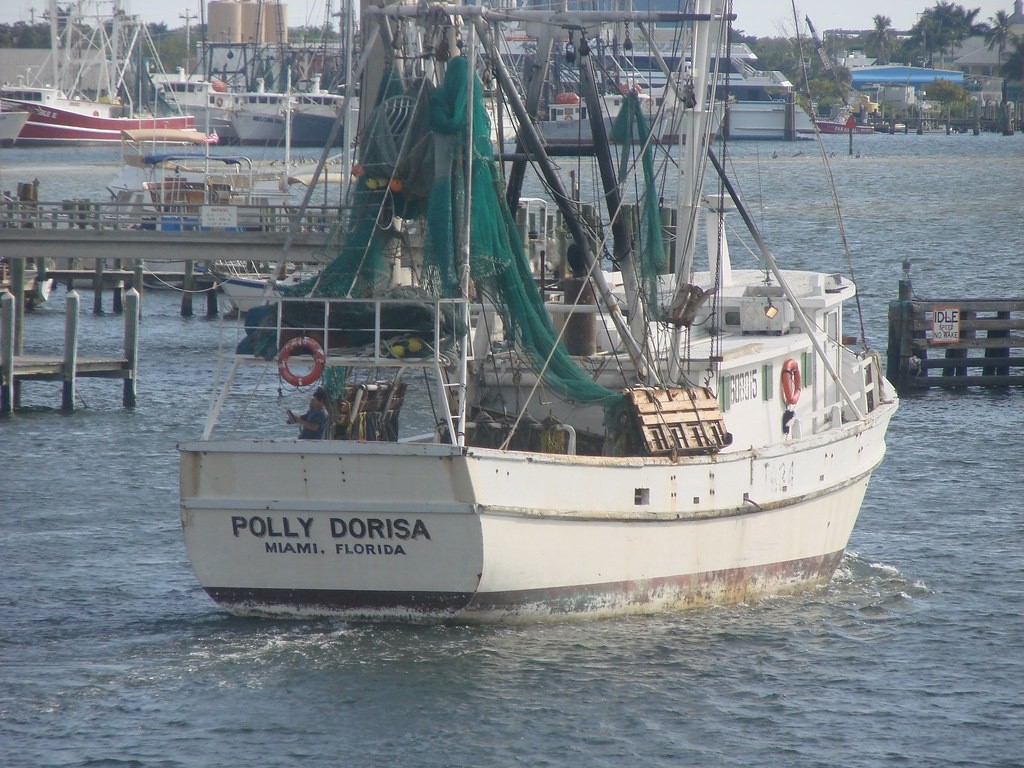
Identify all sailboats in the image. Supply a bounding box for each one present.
[1,0,898,629]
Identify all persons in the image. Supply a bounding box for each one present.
[294,391,329,439]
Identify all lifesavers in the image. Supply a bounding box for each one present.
[780,358,803,407]
[275,334,328,387]
[564,114,573,121]
[216,97,224,108]
